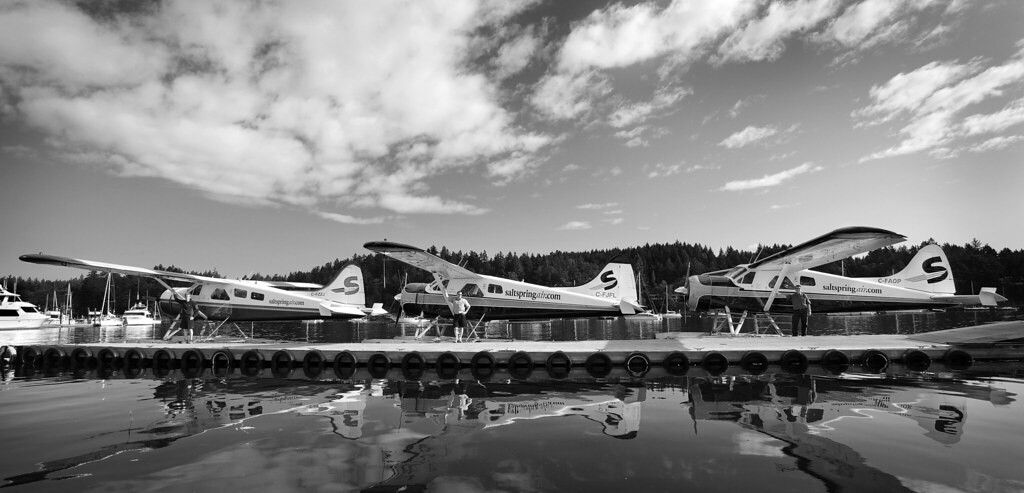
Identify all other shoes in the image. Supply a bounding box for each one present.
[453,340,457,343]
[458,340,461,343]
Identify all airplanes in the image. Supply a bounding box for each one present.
[361,240,646,340]
[19,250,373,337]
[677,226,1008,334]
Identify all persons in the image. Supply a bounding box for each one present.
[174,293,198,344]
[790,284,812,337]
[796,371,817,418]
[453,381,472,421]
[452,291,471,343]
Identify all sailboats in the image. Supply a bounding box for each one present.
[43,269,166,328]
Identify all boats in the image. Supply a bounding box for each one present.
[0,285,50,332]
